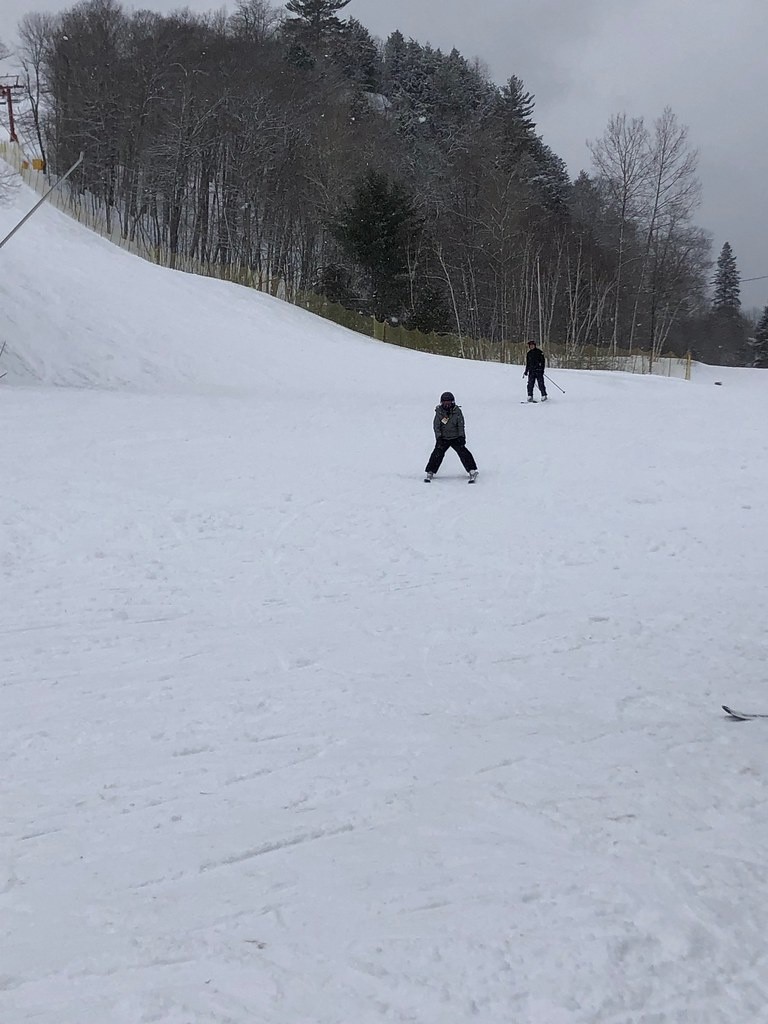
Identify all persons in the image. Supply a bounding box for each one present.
[524,341,547,402]
[424,392,479,485]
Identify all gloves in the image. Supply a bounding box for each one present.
[524,372,528,376]
[458,437,466,446]
[437,436,444,446]
[541,370,544,375]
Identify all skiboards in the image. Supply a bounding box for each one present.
[423,473,477,485]
[520,399,550,405]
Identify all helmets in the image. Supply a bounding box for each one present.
[440,392,455,410]
[527,341,536,345]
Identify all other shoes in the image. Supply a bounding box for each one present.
[527,395,533,401]
[426,471,433,479]
[469,470,480,476]
[541,395,547,401]
